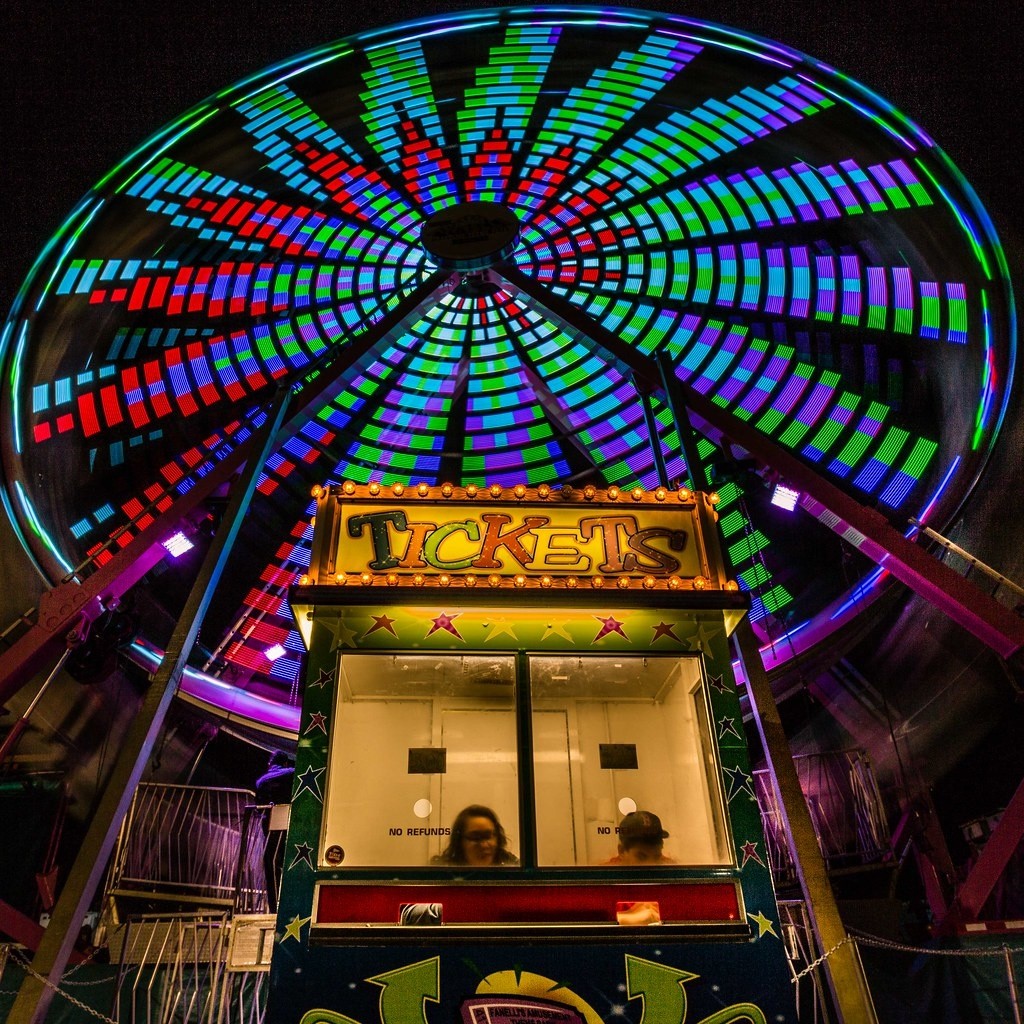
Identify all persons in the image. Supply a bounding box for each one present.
[601,811,678,924]
[401,805,520,926]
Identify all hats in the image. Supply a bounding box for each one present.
[619,810,670,839]
